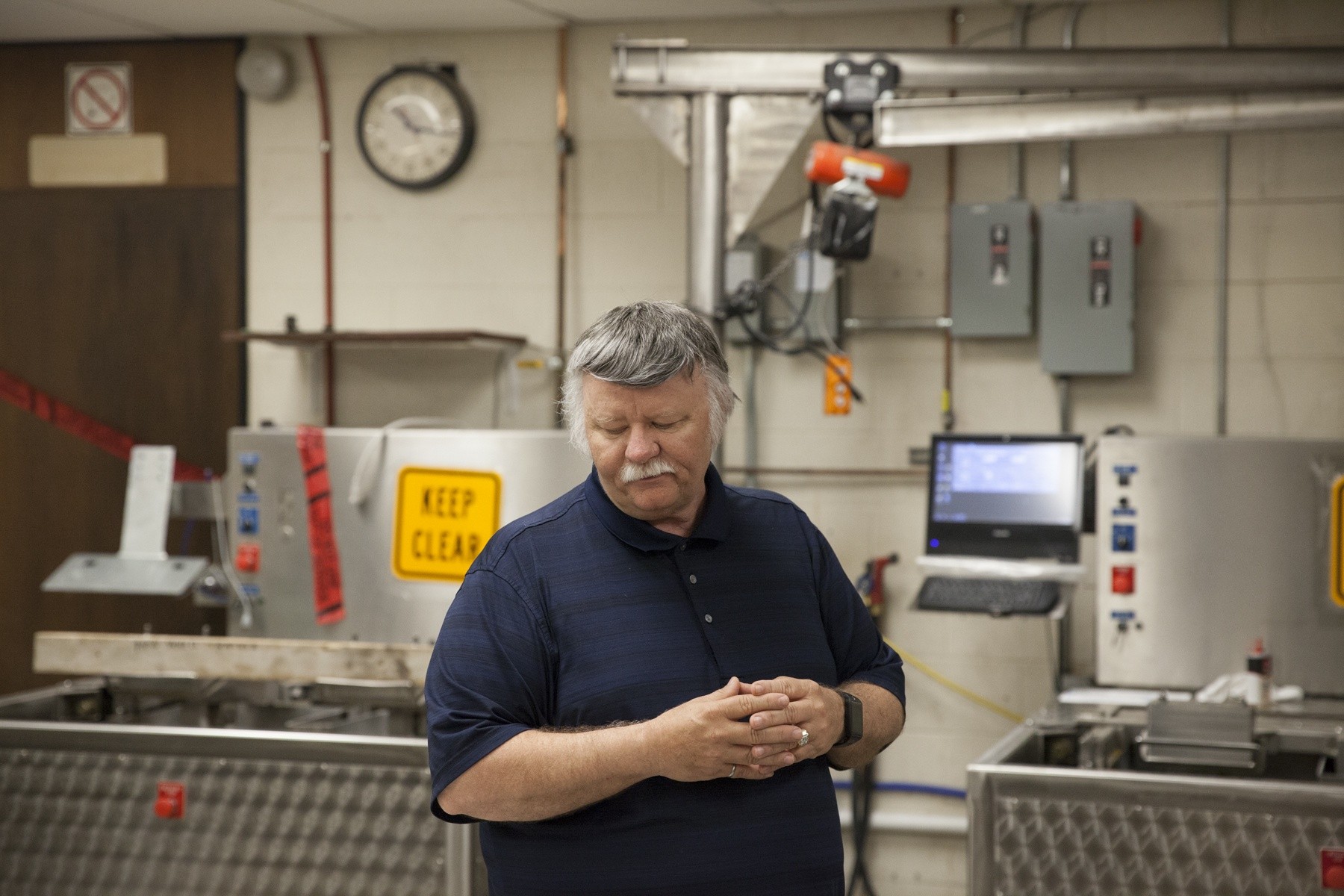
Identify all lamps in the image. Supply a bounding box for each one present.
[235,46,294,103]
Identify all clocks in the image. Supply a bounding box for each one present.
[356,62,475,191]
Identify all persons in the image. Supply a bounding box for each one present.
[426,299,905,896]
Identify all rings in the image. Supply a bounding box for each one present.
[728,764,736,778]
[797,729,809,746]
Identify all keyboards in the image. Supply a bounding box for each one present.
[918,577,1060,614]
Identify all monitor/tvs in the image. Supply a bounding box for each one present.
[923,431,1085,560]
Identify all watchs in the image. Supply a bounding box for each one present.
[831,689,863,749]
